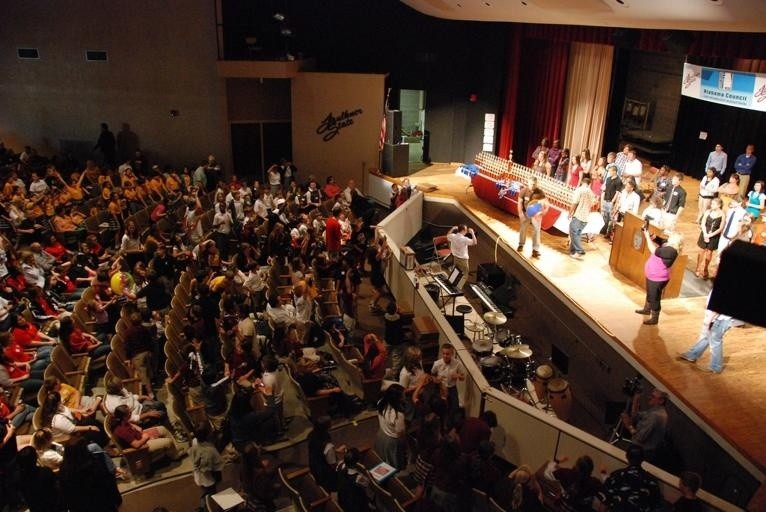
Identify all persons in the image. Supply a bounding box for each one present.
[503,456,608,509]
[532,138,592,188]
[635,228,683,325]
[694,143,765,280]
[517,176,549,256]
[592,144,642,243]
[567,177,594,258]
[638,162,686,231]
[602,444,661,511]
[2,137,499,512]
[669,471,712,511]
[618,387,668,465]
[677,288,743,373]
[91,122,136,163]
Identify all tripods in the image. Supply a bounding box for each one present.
[606,397,641,447]
[517,370,539,410]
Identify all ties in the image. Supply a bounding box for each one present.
[665,186,676,212]
[723,211,736,238]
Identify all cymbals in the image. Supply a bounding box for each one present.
[465,321,486,332]
[500,343,533,359]
[483,311,508,325]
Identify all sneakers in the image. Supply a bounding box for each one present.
[518,246,522,251]
[533,250,540,257]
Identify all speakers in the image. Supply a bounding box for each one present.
[388,110,402,144]
[477,262,506,311]
[15,48,41,59]
[85,50,109,62]
[382,142,410,178]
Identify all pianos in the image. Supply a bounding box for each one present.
[432,272,465,296]
[469,280,518,319]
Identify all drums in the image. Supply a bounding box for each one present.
[495,329,522,348]
[495,352,535,388]
[479,356,505,377]
[471,338,493,361]
[547,378,574,422]
[535,364,555,404]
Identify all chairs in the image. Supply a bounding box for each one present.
[0,166,592,512]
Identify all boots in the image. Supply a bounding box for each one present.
[635,302,651,315]
[643,310,659,325]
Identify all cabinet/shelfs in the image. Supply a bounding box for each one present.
[219,60,298,181]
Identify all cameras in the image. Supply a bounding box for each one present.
[622,372,644,396]
[642,219,650,231]
